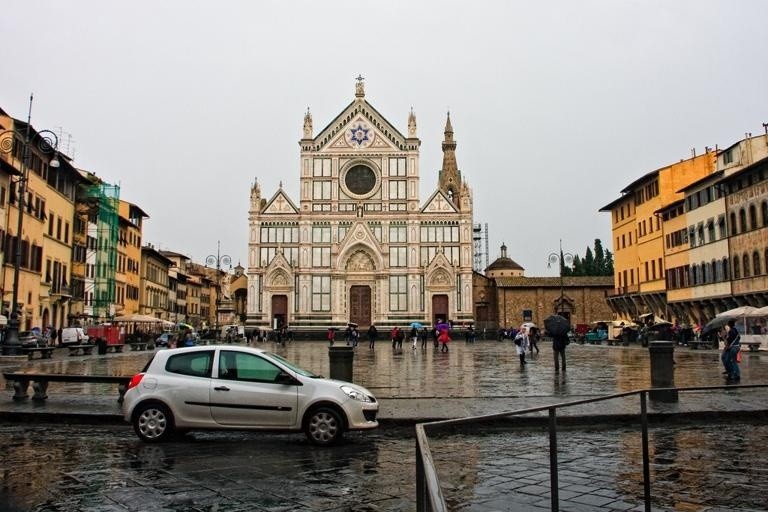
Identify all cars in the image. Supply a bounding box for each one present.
[55,328,89,344]
[17,331,48,348]
[155,333,170,347]
[122,345,379,445]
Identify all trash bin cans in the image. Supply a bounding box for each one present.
[328,346,353,384]
[649,340,676,388]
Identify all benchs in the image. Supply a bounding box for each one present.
[68,345,95,356]
[4,373,132,403]
[688,341,712,350]
[24,347,55,361]
[606,339,621,346]
[107,344,125,353]
[739,343,761,352]
[130,343,147,351]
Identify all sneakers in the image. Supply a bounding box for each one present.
[520,361,526,366]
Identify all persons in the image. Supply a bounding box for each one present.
[636,318,720,349]
[31,326,62,347]
[724,321,741,380]
[552,334,569,371]
[134,323,296,348]
[327,318,541,352]
[749,319,762,352]
[719,328,731,374]
[514,325,530,368]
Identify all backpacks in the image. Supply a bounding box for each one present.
[533,328,540,341]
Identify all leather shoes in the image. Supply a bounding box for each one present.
[722,370,740,384]
[530,349,539,354]
[555,366,567,371]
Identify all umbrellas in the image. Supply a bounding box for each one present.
[702,316,737,336]
[542,314,571,338]
[749,306,768,317]
[715,305,765,336]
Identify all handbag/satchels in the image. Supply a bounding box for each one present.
[565,335,570,345]
[737,351,742,363]
[724,346,732,352]
[514,339,522,346]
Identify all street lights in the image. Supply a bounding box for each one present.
[204,242,232,339]
[547,239,575,314]
[0,93,60,349]
